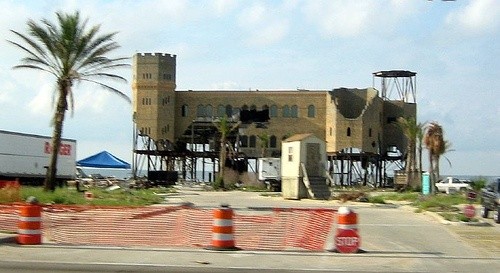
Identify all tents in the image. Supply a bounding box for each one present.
[75,150,132,179]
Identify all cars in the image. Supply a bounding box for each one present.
[480,178,500,223]
[434,177,469,195]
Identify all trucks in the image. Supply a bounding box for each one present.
[0,130,77,187]
